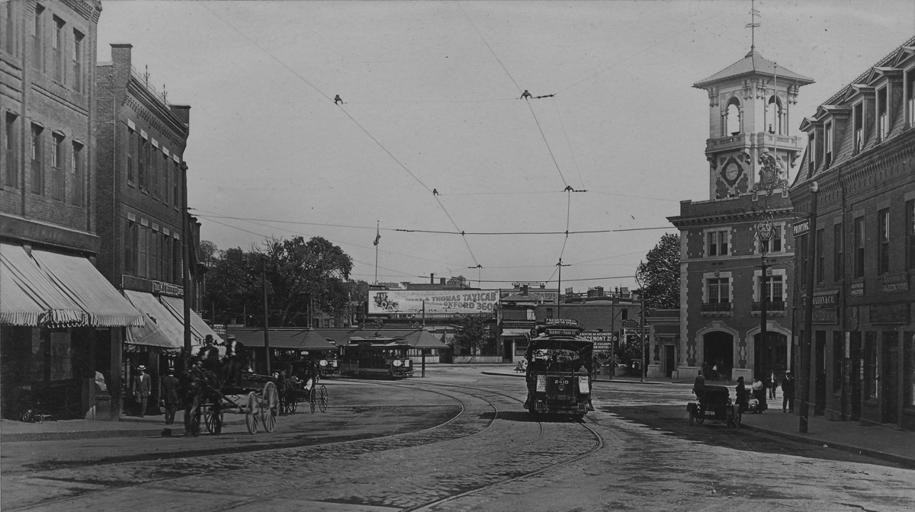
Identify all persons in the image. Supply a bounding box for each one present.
[197,333,220,383]
[693,369,705,400]
[734,374,747,426]
[750,375,764,394]
[161,367,181,425]
[781,369,796,414]
[517,360,521,373]
[130,365,152,417]
[688,389,699,426]
[219,333,246,384]
[767,368,779,400]
[521,360,526,373]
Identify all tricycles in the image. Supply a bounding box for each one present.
[687,386,744,430]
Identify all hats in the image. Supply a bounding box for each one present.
[227,334,235,339]
[205,335,213,340]
[137,365,146,371]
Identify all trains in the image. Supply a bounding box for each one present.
[338,340,413,381]
[524,335,595,422]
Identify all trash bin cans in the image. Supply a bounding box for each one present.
[95,395,111,421]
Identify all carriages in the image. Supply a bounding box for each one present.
[161,367,281,436]
[272,359,328,414]
